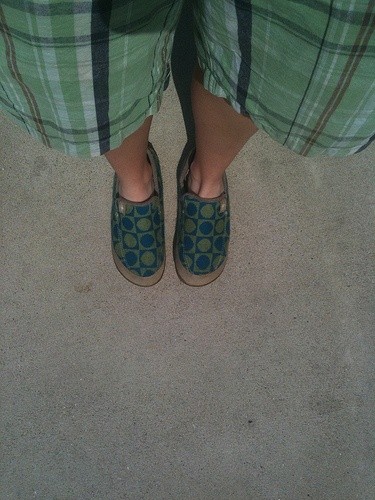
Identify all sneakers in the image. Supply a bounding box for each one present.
[172,147,231,288]
[111,139,167,287]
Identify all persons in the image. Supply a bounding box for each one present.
[1,0,375,286]
